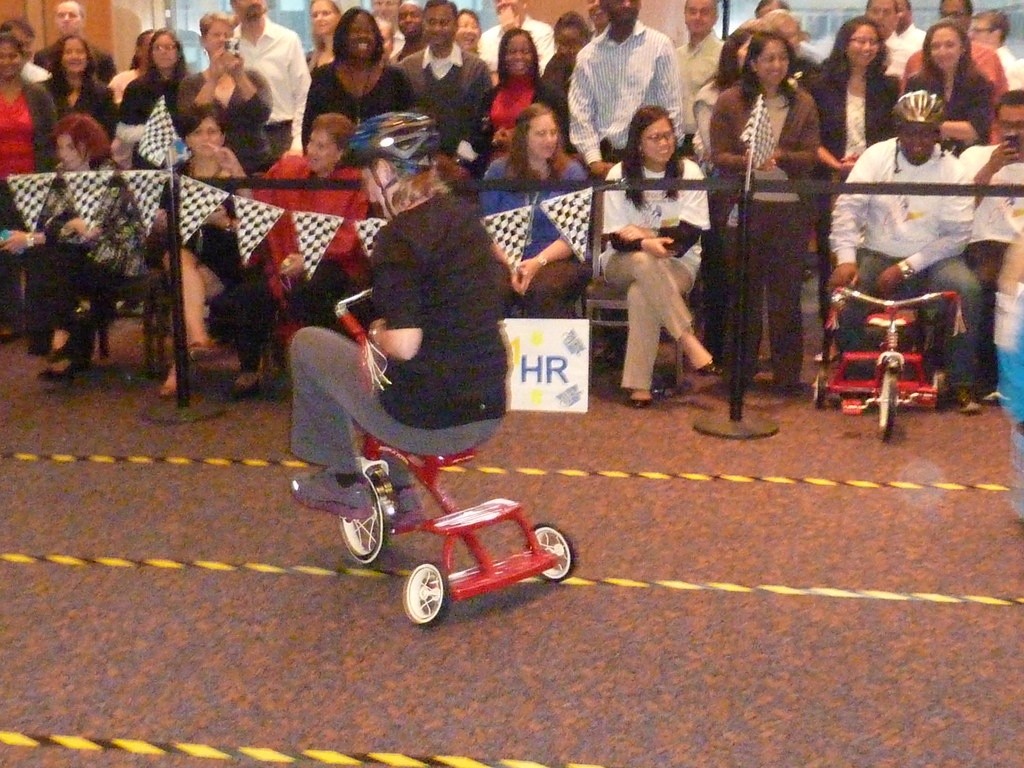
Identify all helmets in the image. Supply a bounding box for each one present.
[892,90,945,129]
[343,110,441,168]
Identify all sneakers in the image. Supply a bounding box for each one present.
[290,468,375,518]
[392,486,422,526]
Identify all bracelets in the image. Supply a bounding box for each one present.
[369,321,383,342]
[534,250,551,267]
[24,231,37,249]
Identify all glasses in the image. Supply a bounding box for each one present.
[153,44,176,51]
[643,131,672,142]
[849,36,879,46]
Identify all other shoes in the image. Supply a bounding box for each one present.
[628,395,654,409]
[188,341,221,361]
[227,377,259,399]
[696,356,723,376]
[779,383,804,398]
[957,392,983,413]
[159,385,176,398]
[36,359,83,381]
[47,331,79,363]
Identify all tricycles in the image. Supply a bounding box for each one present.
[332,287,581,632]
[811,286,963,441]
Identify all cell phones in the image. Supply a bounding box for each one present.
[665,244,683,251]
[1004,132,1020,155]
[224,39,240,56]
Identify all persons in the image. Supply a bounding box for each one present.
[826,91,986,412]
[2,113,151,382]
[959,90,1023,405]
[283,112,511,521]
[599,107,727,406]
[712,36,823,399]
[1,1,1023,351]
[153,111,372,399]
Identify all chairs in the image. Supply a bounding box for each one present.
[584,191,688,388]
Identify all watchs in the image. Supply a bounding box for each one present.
[897,260,915,279]
